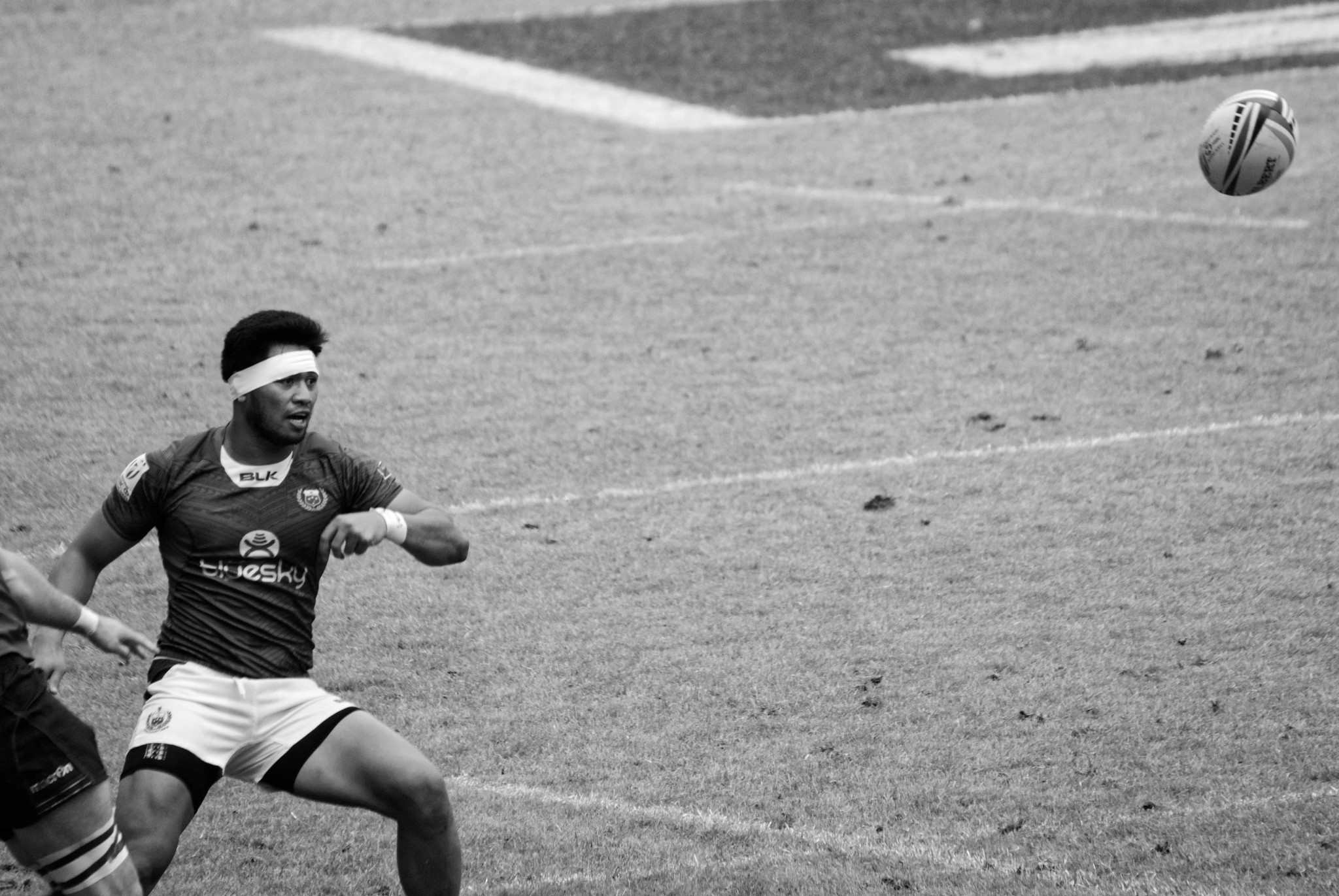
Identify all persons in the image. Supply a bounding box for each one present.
[0,547,160,896]
[29,310,470,896]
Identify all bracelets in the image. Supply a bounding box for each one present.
[71,604,98,638]
[370,506,407,546]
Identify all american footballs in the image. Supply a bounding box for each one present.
[1196,89,1301,199]
